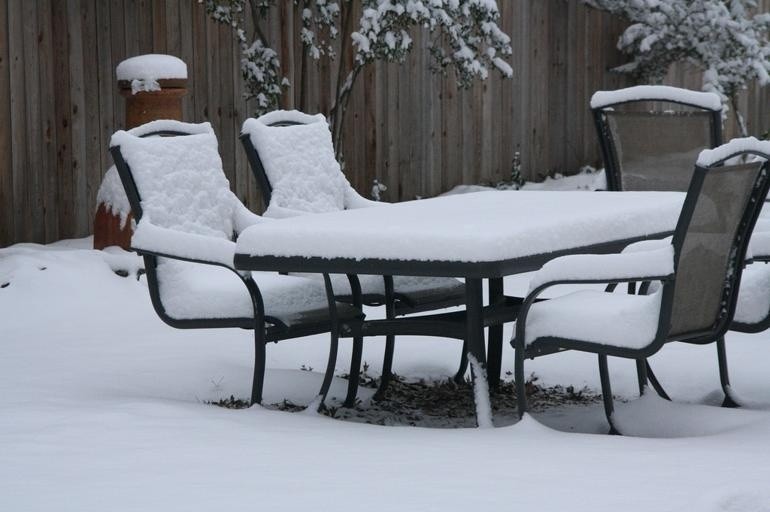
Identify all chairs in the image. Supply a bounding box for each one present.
[714,261,770,413]
[108,120,365,408]
[512,138,770,433]
[589,89,770,263]
[238,112,475,401]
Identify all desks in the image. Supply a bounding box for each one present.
[234,187,723,427]
